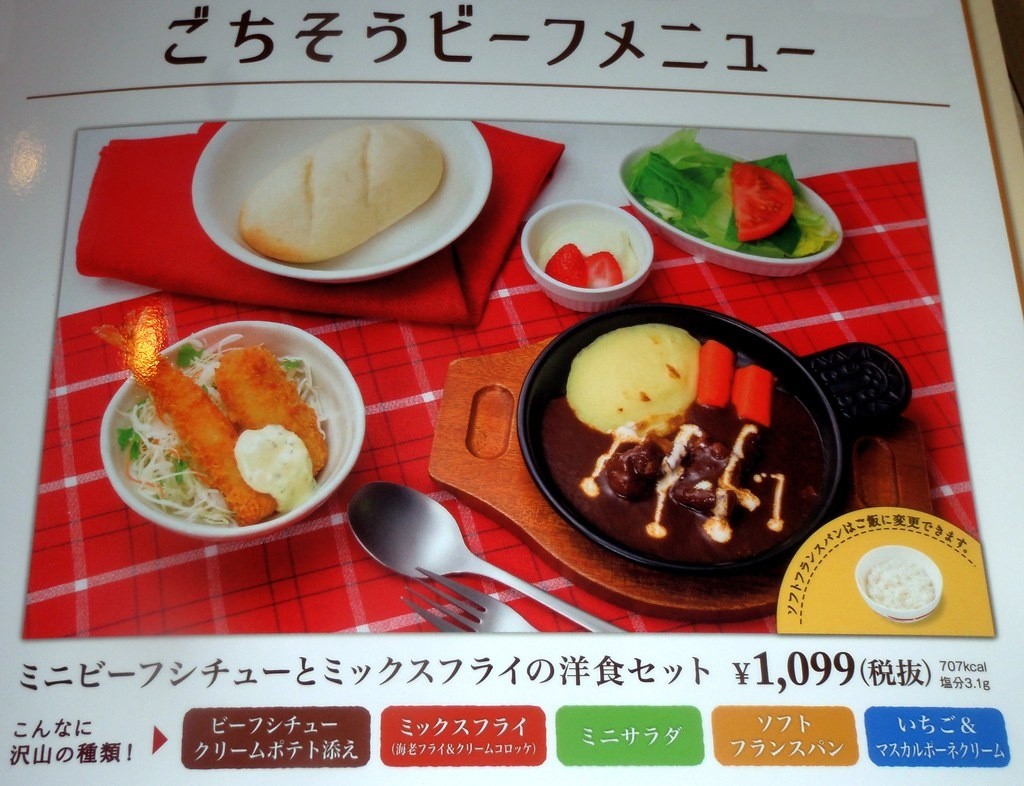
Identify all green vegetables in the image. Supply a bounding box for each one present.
[627,125,839,260]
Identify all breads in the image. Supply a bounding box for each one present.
[242,124,442,263]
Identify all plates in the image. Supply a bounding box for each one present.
[514,302,841,572]
[617,142,844,278]
[191,121,492,284]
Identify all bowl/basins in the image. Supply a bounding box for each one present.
[855,545,944,623]
[100,319,365,539]
[520,200,654,313]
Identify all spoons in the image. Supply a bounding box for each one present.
[347,480,629,634]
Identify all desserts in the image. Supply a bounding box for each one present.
[541,219,636,286]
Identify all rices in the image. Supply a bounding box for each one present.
[863,554,937,610]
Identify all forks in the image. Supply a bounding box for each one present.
[398,567,544,633]
